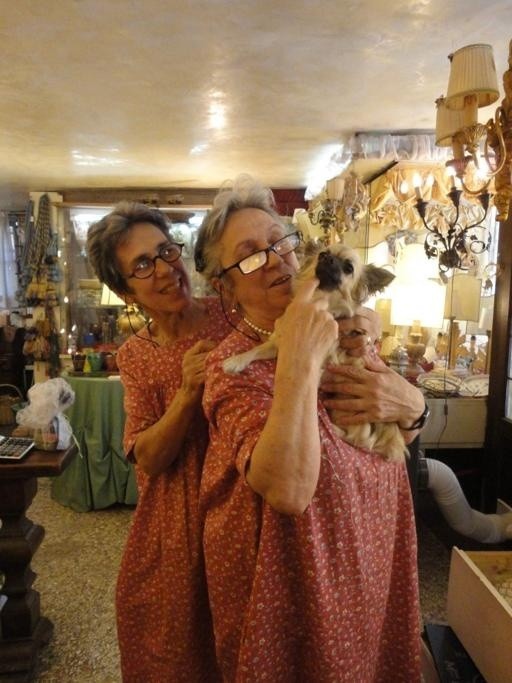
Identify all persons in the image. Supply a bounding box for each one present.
[85,199,376,682]
[194,170,431,682]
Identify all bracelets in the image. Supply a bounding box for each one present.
[399,403,430,431]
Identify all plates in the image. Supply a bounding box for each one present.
[416,372,491,395]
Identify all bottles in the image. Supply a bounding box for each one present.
[101,309,117,344]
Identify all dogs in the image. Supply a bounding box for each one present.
[220,242,412,465]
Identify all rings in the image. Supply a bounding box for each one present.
[367,335,373,345]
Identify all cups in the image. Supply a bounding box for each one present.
[82,332,95,345]
[69,351,120,373]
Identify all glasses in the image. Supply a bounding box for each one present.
[219,229,304,280]
[122,242,186,284]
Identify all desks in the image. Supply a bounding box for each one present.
[60,365,137,513]
[0,423,79,683]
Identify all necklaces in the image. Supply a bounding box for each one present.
[242,316,273,335]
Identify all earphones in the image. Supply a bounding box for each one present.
[116,286,127,296]
[217,283,225,292]
